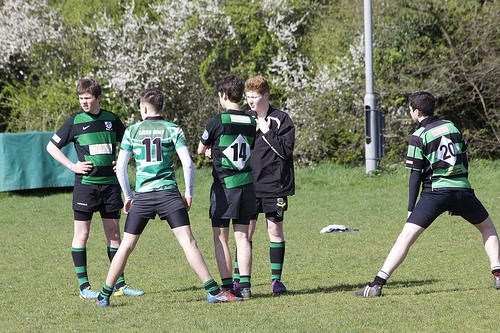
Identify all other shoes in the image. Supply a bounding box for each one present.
[238,286,251,299]
[222,283,239,296]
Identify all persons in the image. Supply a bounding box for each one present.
[354,92,500,298]
[93,89,246,308]
[232,76,296,293]
[46,78,145,300]
[198,76,256,300]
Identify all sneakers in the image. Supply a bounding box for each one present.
[207,289,241,302]
[355,281,382,296]
[96,297,109,307]
[79,288,100,300]
[271,279,286,293]
[112,284,144,296]
[233,281,239,289]
[494,272,500,288]
